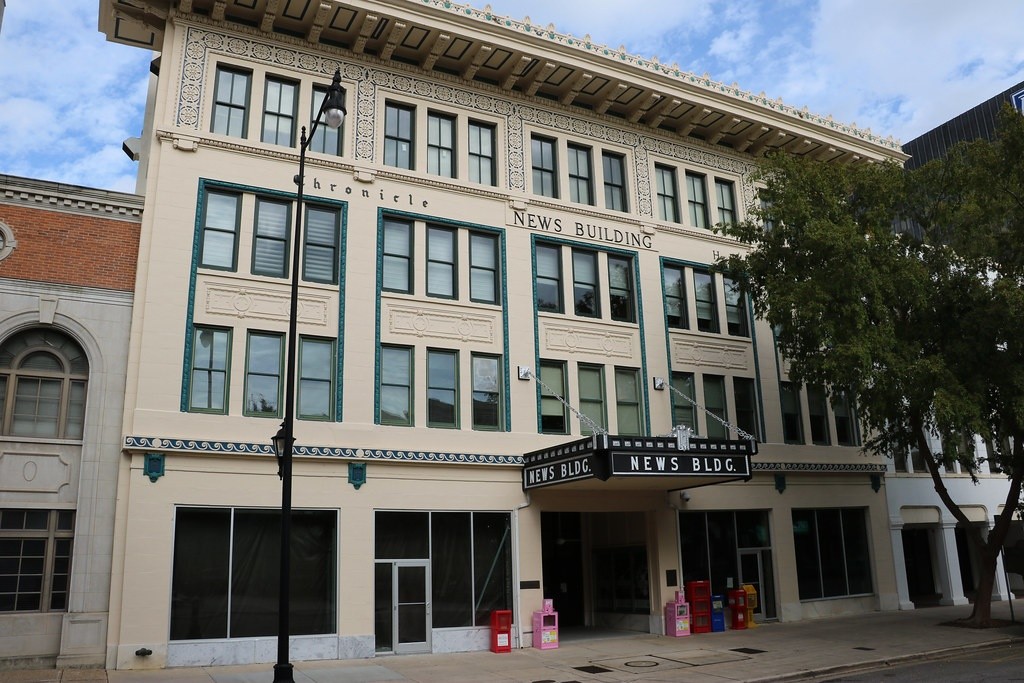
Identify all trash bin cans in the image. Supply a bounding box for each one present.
[711,595,726,632]
[532,599,559,649]
[728,590,748,630]
[739,585,759,628]
[665,590,691,637]
[490,608,512,653]
[683,580,711,633]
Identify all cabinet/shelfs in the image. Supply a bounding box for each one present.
[665,602,689,636]
[492,610,511,653]
[688,581,713,634]
[533,611,558,650]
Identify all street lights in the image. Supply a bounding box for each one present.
[272,68,347,683]
